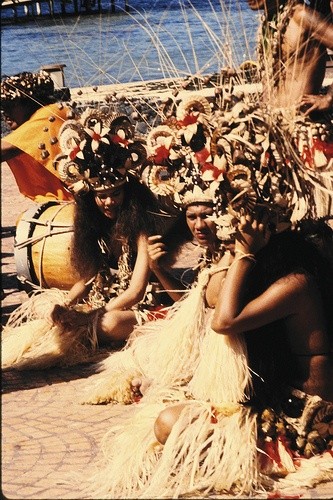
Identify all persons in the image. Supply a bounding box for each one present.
[243,0,333,119]
[0,73,75,191]
[33,181,149,340]
[147,200,333,446]
